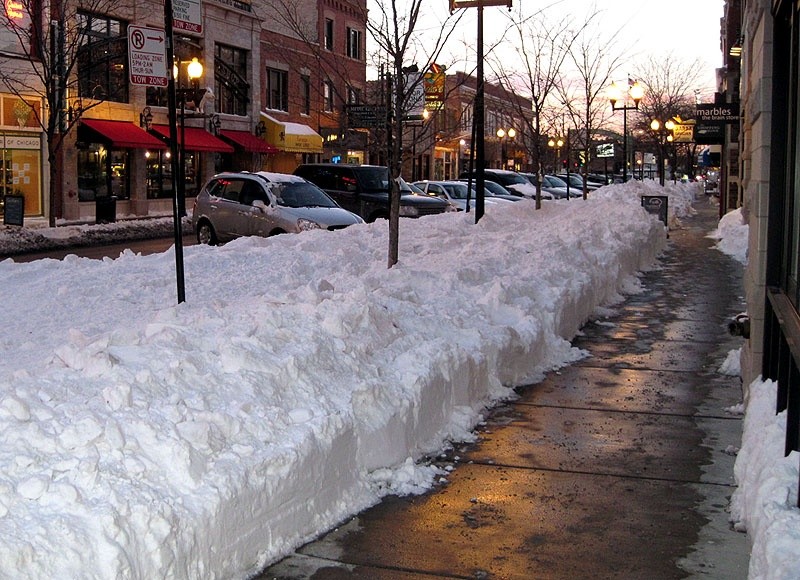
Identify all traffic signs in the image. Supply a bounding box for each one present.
[127,24,170,89]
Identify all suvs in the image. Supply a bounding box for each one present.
[190,170,368,245]
[282,162,453,227]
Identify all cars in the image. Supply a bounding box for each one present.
[401,168,638,210]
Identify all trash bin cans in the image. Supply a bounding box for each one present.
[95,194,117,224]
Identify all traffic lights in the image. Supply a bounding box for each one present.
[562,159,567,169]
[574,162,578,168]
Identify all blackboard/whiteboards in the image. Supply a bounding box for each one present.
[3,194,25,226]
[641,195,668,226]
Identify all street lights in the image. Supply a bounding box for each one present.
[173,54,205,219]
[605,79,646,183]
[547,138,564,174]
[650,118,675,187]
[404,108,429,181]
[497,126,517,170]
